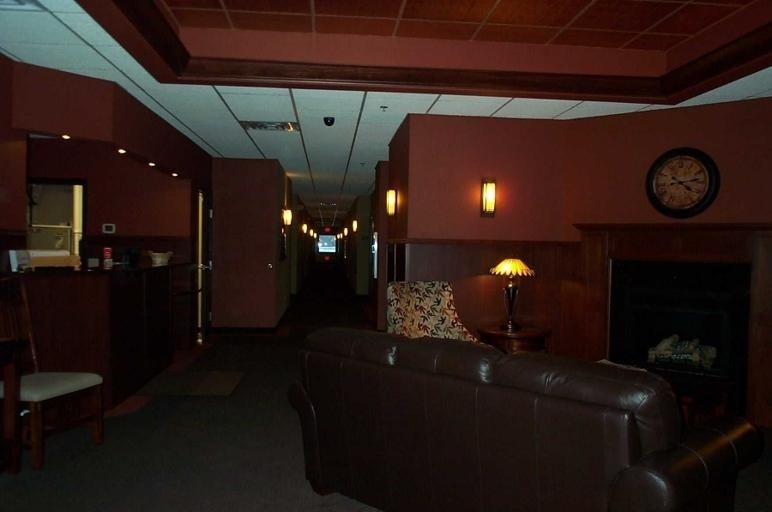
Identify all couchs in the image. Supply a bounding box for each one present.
[287,325,764,511]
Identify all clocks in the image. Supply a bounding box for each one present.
[644,147,722,220]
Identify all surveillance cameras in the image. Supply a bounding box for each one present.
[324,117,335,126]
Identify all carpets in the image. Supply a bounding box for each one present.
[133,368,246,396]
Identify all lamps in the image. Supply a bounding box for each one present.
[479,174,497,218]
[488,258,537,332]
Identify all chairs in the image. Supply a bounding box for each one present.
[386,279,484,345]
[0,271,104,469]
